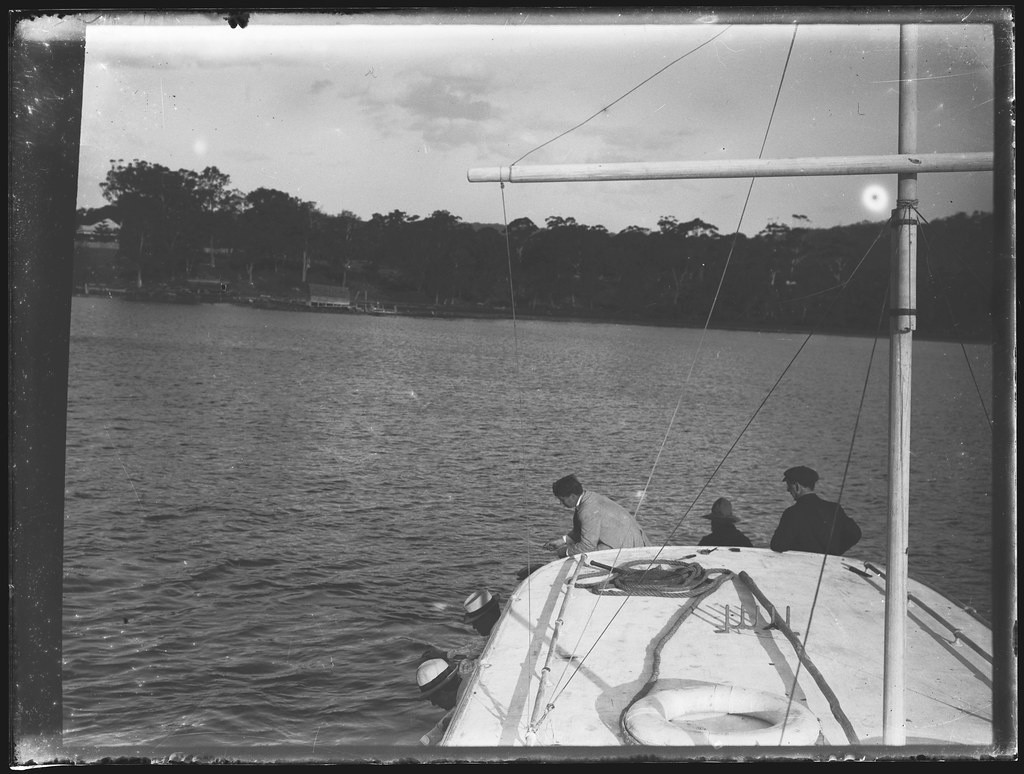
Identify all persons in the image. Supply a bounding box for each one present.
[771,465,862,556]
[543,474,653,558]
[698,498,753,547]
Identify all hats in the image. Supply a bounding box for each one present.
[782,466,819,482]
[698,497,741,523]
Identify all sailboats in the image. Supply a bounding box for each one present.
[417,24,994,746]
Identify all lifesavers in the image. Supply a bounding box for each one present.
[621,683,820,747]
